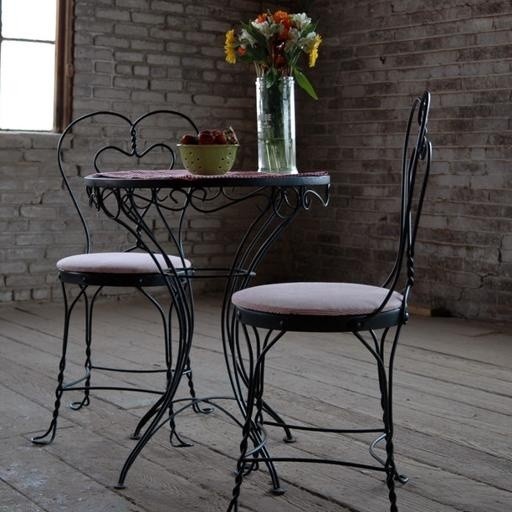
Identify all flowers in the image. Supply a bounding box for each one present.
[225,8,322,171]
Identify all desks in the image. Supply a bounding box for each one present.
[82,170,331,496]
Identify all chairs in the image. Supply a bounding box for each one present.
[30,110,214,448]
[227,90,432,511]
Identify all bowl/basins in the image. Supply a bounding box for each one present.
[176,143,240,174]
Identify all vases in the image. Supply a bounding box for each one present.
[255,77,298,175]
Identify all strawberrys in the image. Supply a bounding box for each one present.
[181,130,230,144]
